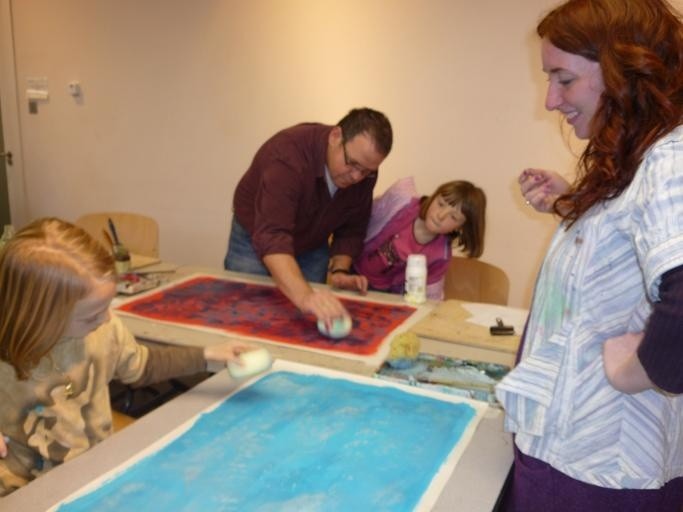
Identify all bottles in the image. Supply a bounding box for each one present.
[226,348,272,378]
[404,254,428,303]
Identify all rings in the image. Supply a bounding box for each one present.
[525,199,530,205]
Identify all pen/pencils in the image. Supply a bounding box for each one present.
[108,217,119,245]
[102,227,114,250]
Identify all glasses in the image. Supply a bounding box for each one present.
[343,144,378,180]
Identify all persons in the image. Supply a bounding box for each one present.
[222,106,392,334]
[488,1,683,512]
[322,176,487,301]
[0,216,260,504]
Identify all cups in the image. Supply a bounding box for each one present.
[111,245,131,275]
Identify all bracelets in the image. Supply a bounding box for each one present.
[330,268,350,276]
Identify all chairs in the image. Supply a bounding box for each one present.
[444,253,509,305]
[75,212,159,266]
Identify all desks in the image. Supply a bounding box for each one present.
[109,266,529,411]
[0,354,516,511]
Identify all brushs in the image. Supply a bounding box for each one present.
[490,317,513,334]
